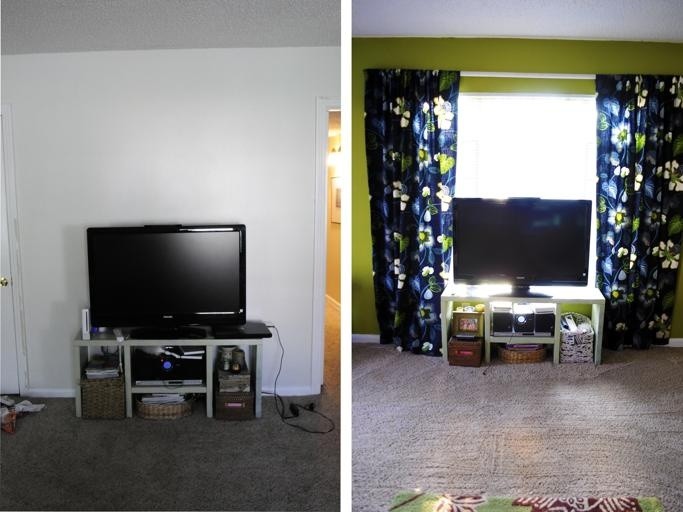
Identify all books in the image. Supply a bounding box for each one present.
[139,392,187,405]
[83,352,122,380]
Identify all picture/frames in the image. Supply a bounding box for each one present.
[452,311,483,338]
[330,176,341,224]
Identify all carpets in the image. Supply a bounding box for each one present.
[389,489,666,512]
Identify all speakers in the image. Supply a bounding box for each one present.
[490,302,513,337]
[530,303,555,337]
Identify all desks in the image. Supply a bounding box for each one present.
[72,320,272,419]
[441,287,606,366]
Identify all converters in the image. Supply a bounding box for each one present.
[290,403,299,417]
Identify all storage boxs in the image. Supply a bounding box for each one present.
[218,363,250,393]
[214,391,254,422]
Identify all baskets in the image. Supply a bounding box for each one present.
[498,345,547,364]
[215,387,254,420]
[135,394,193,420]
[81,379,125,420]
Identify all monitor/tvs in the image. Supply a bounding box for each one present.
[452,197,592,298]
[86,224,247,339]
